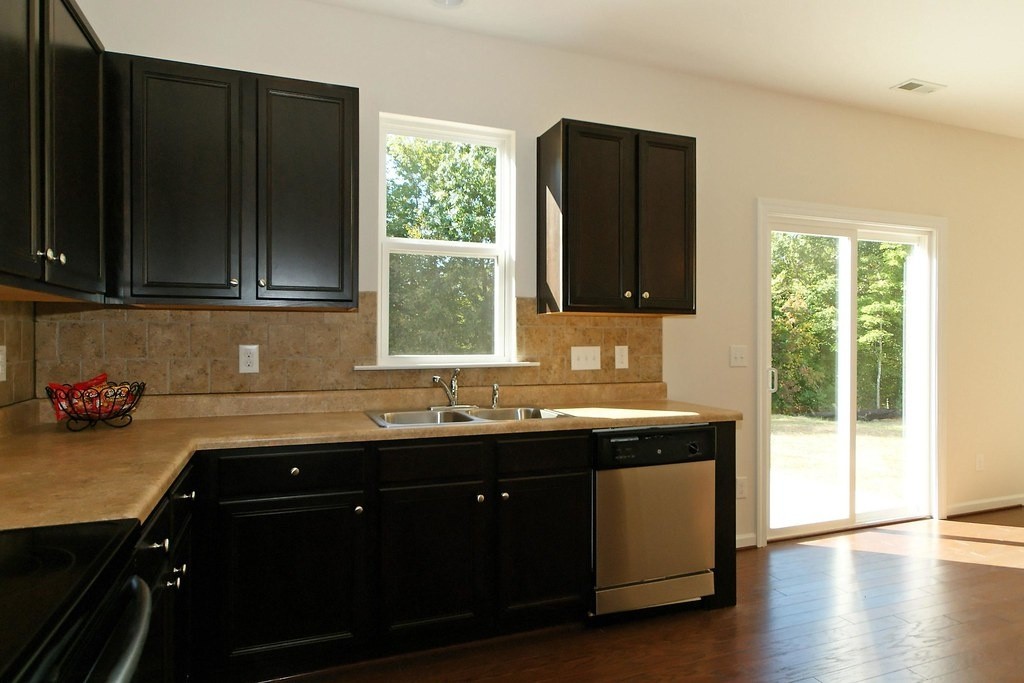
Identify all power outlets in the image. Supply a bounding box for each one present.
[615,346,629,369]
[239,345,259,374]
[570,347,601,370]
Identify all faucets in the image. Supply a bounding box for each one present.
[431,368,461,405]
[491,383,501,409]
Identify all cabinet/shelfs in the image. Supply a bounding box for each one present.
[0,0,360,315]
[131,431,595,683]
[536,118,696,316]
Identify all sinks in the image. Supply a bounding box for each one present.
[465,406,578,423]
[362,403,475,430]
